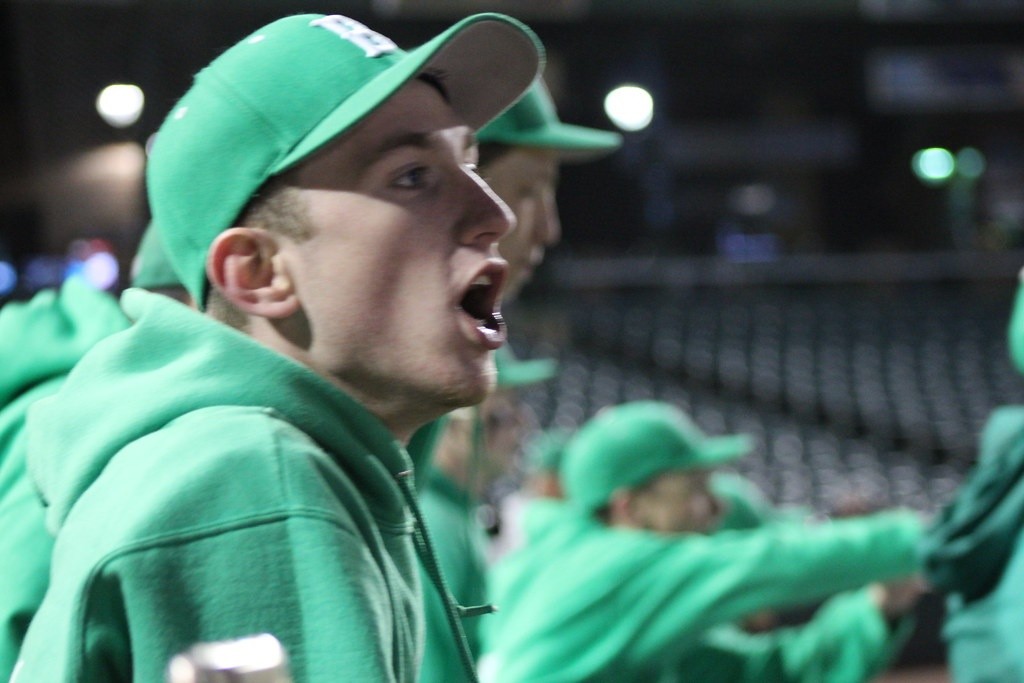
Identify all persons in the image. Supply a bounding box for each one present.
[406,70,1024,683]
[473,399,936,683]
[0,13,547,683]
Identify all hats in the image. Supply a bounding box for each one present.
[523,428,576,472]
[475,76,625,165]
[129,220,182,289]
[146,12,547,313]
[493,346,561,391]
[702,471,774,540]
[558,400,754,521]
